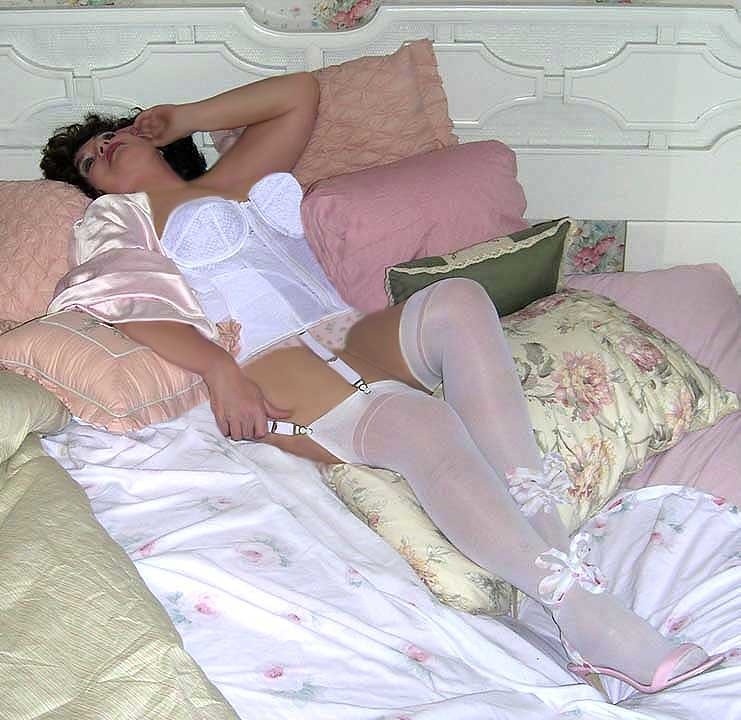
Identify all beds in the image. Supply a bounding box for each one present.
[0,7,740,720]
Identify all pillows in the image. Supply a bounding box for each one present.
[320,287,740,616]
[209,38,460,195]
[0,370,71,465]
[301,140,531,314]
[0,311,213,435]
[384,216,570,318]
[0,181,92,334]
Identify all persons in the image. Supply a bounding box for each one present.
[39,71,728,700]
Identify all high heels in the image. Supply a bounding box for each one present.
[568,641,724,703]
